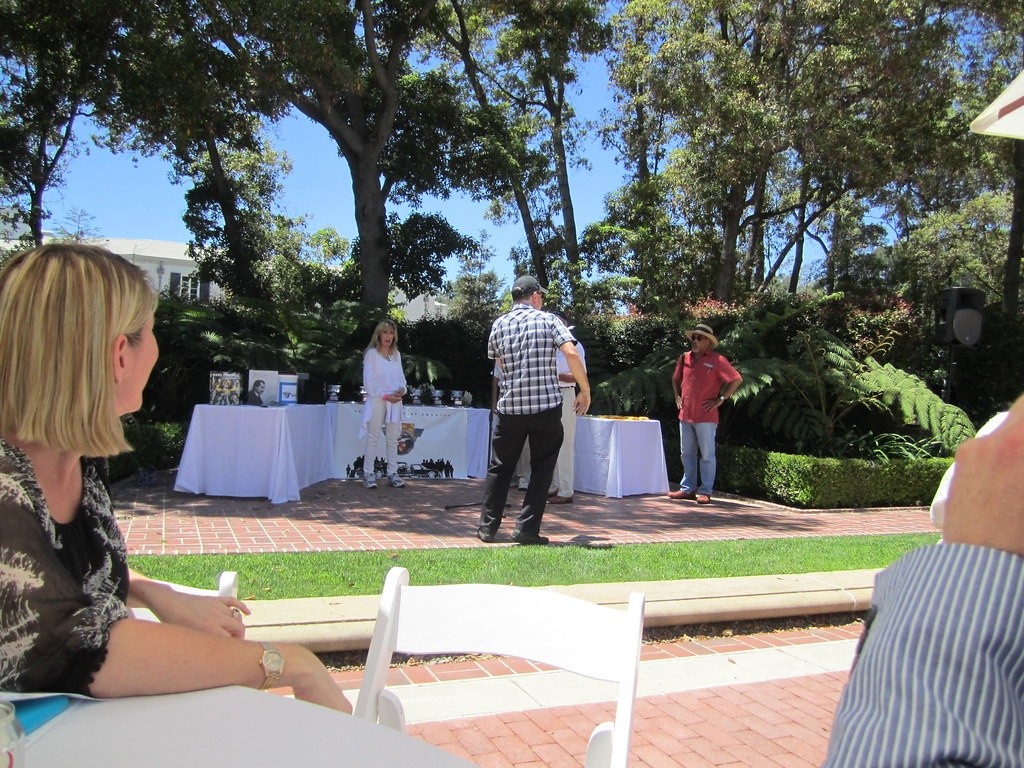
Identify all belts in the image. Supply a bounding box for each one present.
[558,385,573,389]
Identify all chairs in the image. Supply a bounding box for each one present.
[138,563,647,768]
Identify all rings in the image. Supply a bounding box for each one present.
[233,609,239,617]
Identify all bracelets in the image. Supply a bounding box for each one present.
[384,394,387,400]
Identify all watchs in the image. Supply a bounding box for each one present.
[720,395,726,401]
[259,642,285,689]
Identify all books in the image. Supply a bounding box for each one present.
[209,369,298,406]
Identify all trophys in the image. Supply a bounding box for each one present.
[452,391,464,408]
[432,390,444,407]
[326,385,345,404]
[411,390,424,406]
[360,386,367,404]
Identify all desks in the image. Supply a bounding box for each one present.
[172,404,672,505]
[14,686,473,768]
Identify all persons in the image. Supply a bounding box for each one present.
[547,311,587,503]
[476,275,590,545]
[248,380,265,405]
[817,392,1024,768]
[491,360,531,490]
[0,243,353,714]
[667,324,743,503]
[360,319,408,488]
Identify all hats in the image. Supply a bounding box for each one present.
[512,275,549,300]
[685,324,718,350]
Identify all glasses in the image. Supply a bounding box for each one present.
[690,335,708,341]
[532,292,544,298]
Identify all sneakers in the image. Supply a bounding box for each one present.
[547,491,558,497]
[363,473,377,489]
[510,529,549,545]
[518,478,529,491]
[667,490,695,500]
[547,495,573,504]
[478,528,494,542]
[697,493,710,504]
[509,477,518,485]
[388,472,406,488]
